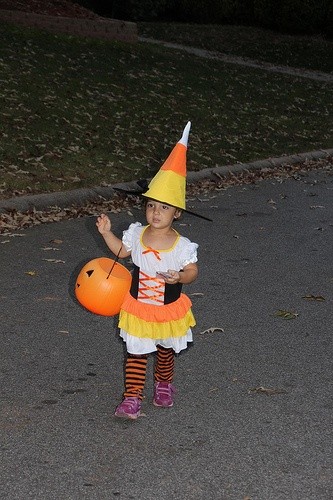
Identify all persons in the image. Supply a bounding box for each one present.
[96,122,199,418]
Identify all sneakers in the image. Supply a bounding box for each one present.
[152,382,176,408]
[115,397,142,419]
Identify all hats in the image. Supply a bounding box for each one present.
[112,121,214,222]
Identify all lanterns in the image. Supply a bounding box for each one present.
[74,257,132,317]
